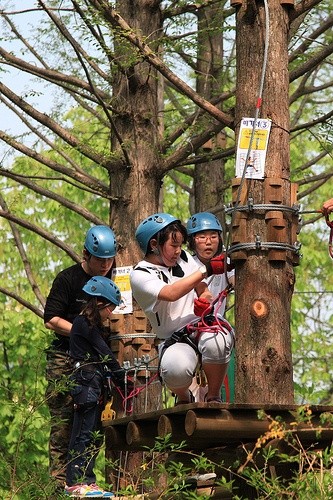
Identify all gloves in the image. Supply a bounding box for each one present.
[194,298,214,317]
[121,376,138,399]
[206,252,230,275]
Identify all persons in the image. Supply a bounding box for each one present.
[64,276,134,498]
[44,226,117,479]
[129,213,235,404]
[186,212,235,400]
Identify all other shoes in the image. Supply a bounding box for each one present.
[64,484,103,498]
[89,483,115,498]
[174,389,195,407]
[203,393,223,403]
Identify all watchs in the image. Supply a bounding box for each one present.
[198,266,207,279]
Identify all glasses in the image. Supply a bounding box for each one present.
[193,235,219,243]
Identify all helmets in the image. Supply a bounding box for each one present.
[186,212,223,236]
[135,213,181,256]
[84,225,118,259]
[82,276,121,307]
